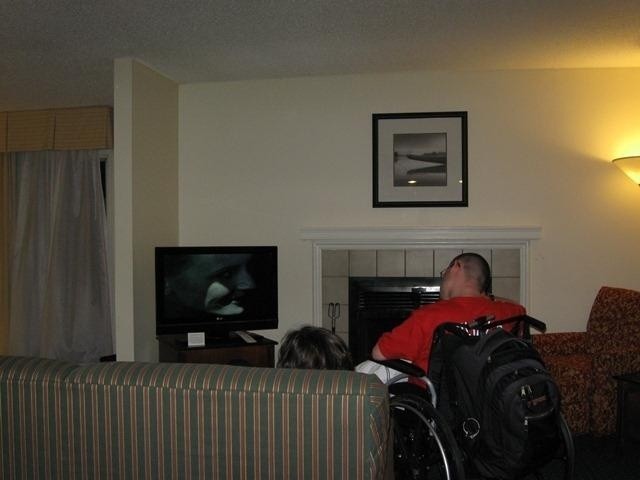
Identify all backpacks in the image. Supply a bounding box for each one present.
[428,315,576,480]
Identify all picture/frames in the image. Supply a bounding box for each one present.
[372,112,468,208]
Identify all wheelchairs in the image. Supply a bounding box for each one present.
[369,314,577,479]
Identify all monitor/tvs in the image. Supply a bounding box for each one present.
[155,245,278,340]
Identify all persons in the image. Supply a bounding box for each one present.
[353,251,529,389]
[275,324,354,370]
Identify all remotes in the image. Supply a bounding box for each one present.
[234,331,257,344]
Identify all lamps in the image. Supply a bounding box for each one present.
[612,156,640,186]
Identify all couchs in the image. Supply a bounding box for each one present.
[1,357,395,480]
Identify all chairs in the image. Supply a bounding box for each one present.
[530,286,640,439]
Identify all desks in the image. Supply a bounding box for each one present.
[156,332,278,368]
[611,372,640,457]
